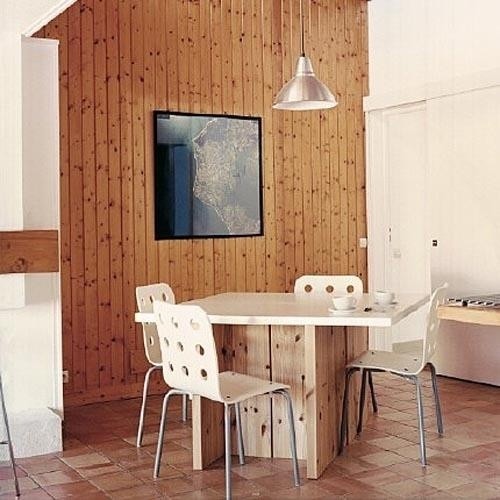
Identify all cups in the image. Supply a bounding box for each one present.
[332,295,357,309]
[374,290,398,304]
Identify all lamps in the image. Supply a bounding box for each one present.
[272,0,338,111]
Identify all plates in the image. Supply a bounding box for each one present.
[373,302,399,307]
[328,307,358,313]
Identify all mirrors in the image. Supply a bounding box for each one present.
[154,111,264,241]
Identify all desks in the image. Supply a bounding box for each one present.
[439,304,500,327]
